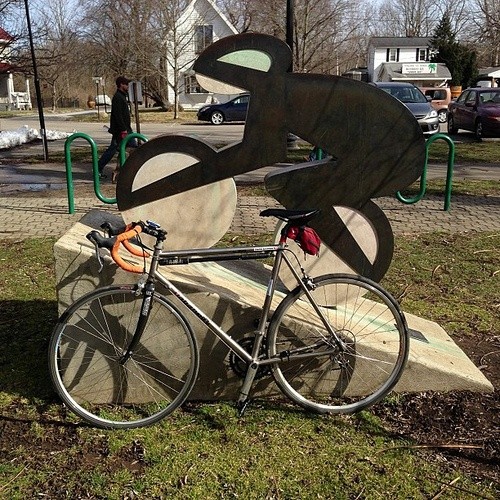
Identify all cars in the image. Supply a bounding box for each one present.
[197,94,251,125]
[447,88,500,140]
[95,95,111,106]
[365,82,440,142]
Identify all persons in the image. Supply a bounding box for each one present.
[98,77,136,178]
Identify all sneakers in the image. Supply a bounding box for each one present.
[92,169,106,178]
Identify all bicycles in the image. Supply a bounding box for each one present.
[46,208,409,432]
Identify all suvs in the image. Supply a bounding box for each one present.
[393,87,453,123]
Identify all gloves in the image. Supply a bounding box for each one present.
[121,130,127,138]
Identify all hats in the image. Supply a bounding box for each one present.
[116,76,132,84]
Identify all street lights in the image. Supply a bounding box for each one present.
[334,48,341,75]
